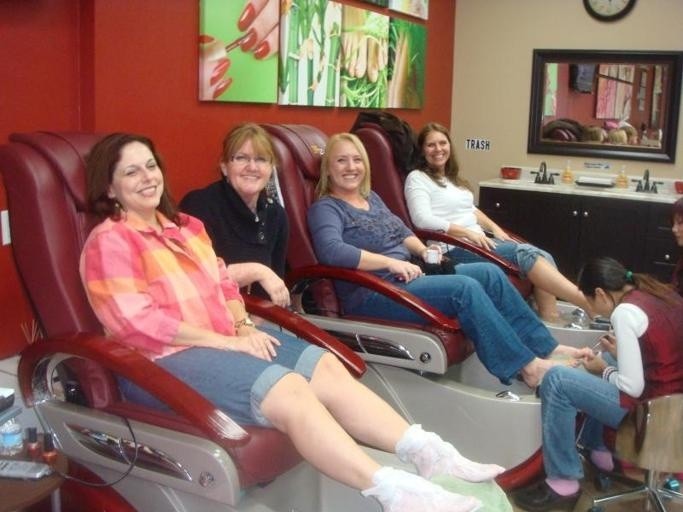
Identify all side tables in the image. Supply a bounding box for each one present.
[0,437,69,512]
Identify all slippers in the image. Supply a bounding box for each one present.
[517,479,583,512]
[582,447,616,494]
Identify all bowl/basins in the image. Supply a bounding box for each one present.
[674,182,683,194]
[501,167,521,178]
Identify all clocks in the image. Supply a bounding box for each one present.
[582,0,637,23]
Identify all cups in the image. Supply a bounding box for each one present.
[426,249,438,263]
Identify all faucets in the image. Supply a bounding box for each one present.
[540,161,548,184]
[643,168,651,193]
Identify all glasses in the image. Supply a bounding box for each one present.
[228,152,270,166]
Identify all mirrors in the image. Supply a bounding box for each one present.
[527,48,683,165]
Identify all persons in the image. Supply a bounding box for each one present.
[665,195,683,296]
[619,126,640,146]
[339,7,387,84]
[403,124,597,327]
[79,132,504,512]
[513,257,682,512]
[640,123,660,147]
[308,132,592,408]
[580,125,605,144]
[175,121,297,336]
[606,129,629,145]
[200,0,279,98]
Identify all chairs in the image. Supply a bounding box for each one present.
[589,394,682,512]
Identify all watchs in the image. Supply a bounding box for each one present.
[234,318,255,334]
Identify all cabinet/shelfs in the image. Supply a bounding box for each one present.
[478,167,683,293]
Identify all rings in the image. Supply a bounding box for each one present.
[408,271,413,274]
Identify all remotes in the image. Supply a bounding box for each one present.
[0,461,48,478]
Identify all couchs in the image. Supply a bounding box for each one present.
[344,127,608,350]
[0,131,513,512]
[257,124,611,491]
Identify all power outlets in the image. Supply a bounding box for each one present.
[0,210,12,246]
